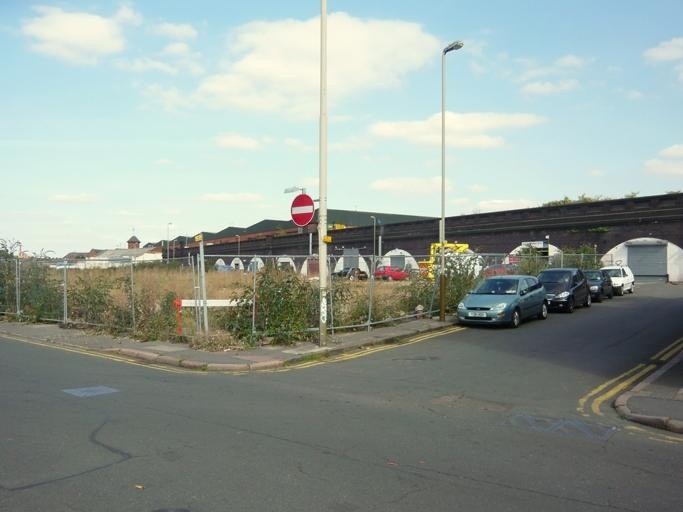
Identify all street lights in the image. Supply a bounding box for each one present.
[439,36,464,319]
[166,223,173,265]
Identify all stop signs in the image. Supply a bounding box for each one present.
[290,194,315,227]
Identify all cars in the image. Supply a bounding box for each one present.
[582,269,613,303]
[456,274,549,328]
[331,267,368,282]
[537,268,592,313]
[376,266,410,281]
[600,265,635,296]
